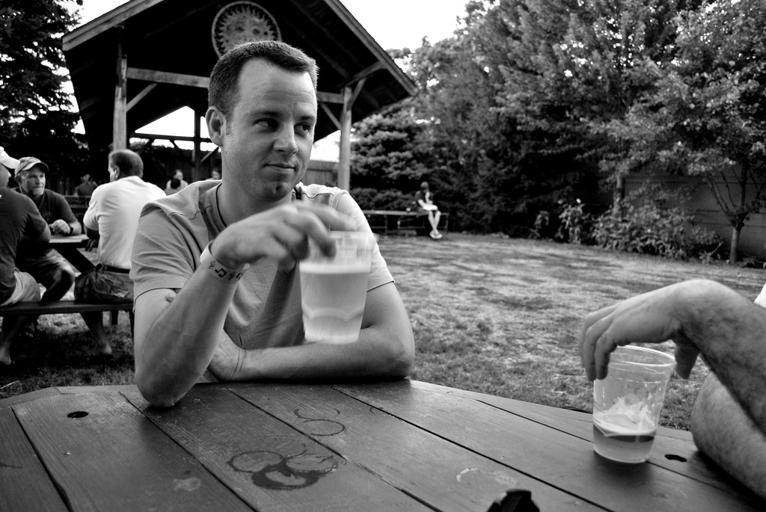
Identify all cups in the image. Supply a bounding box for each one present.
[297,230,379,344]
[590,345,677,464]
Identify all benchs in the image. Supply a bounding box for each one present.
[1,300,134,360]
[360,209,451,239]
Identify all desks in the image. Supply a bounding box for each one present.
[0,380,766,511]
[0,234,117,328]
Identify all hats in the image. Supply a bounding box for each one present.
[1,146,22,170]
[15,155,49,179]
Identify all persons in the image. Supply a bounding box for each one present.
[164,168,220,196]
[127,39,416,409]
[72,173,98,195]
[10,156,80,337]
[82,148,168,358]
[414,181,443,240]
[0,146,52,367]
[578,278,766,500]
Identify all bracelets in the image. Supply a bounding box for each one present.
[200,240,250,283]
